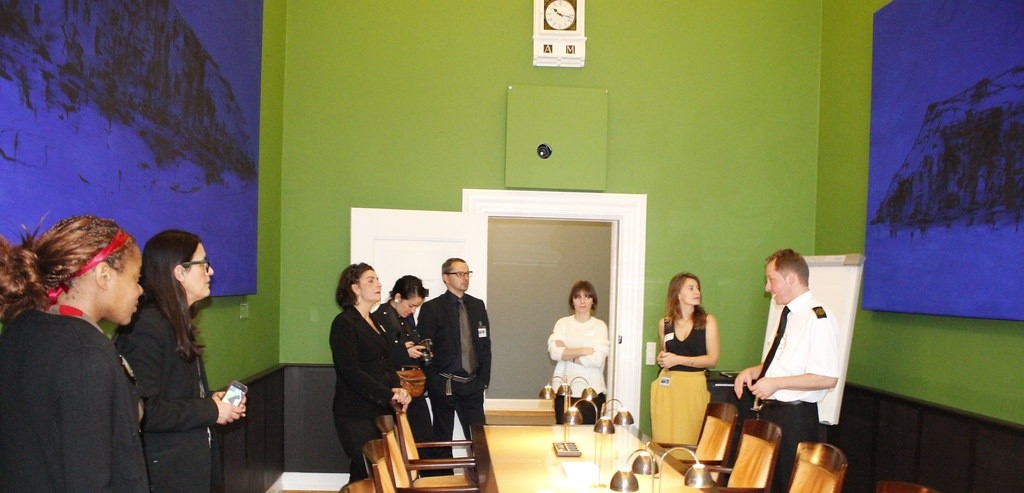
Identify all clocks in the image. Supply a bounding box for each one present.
[532,0,586,68]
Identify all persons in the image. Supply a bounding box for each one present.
[418,258,492,474]
[657,273,720,378]
[733,249,840,493]
[0,214,149,493]
[373,275,441,477]
[112,229,246,493]
[329,262,411,485]
[547,280,610,425]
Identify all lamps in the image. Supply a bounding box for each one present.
[537,373,716,493]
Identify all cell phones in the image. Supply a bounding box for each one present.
[221,380,247,407]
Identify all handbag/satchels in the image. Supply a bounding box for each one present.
[397,364,427,397]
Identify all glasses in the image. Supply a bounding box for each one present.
[573,294,590,300]
[446,271,473,277]
[182,258,211,272]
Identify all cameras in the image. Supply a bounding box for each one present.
[416,339,433,366]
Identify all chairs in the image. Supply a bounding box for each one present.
[656,401,937,493]
[338,399,479,493]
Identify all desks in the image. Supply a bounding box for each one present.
[484,424,704,493]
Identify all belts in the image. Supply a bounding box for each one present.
[438,371,478,396]
[764,399,817,406]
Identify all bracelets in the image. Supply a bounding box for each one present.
[573,358,575,362]
[691,360,693,367]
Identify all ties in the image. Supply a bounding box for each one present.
[756,306,790,384]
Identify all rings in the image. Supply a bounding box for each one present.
[404,393,407,396]
